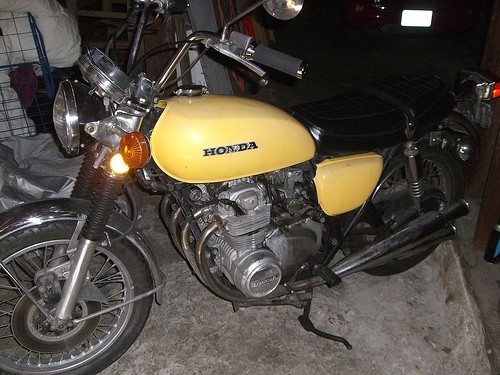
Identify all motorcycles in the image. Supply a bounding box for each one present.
[0,0,500,375]
